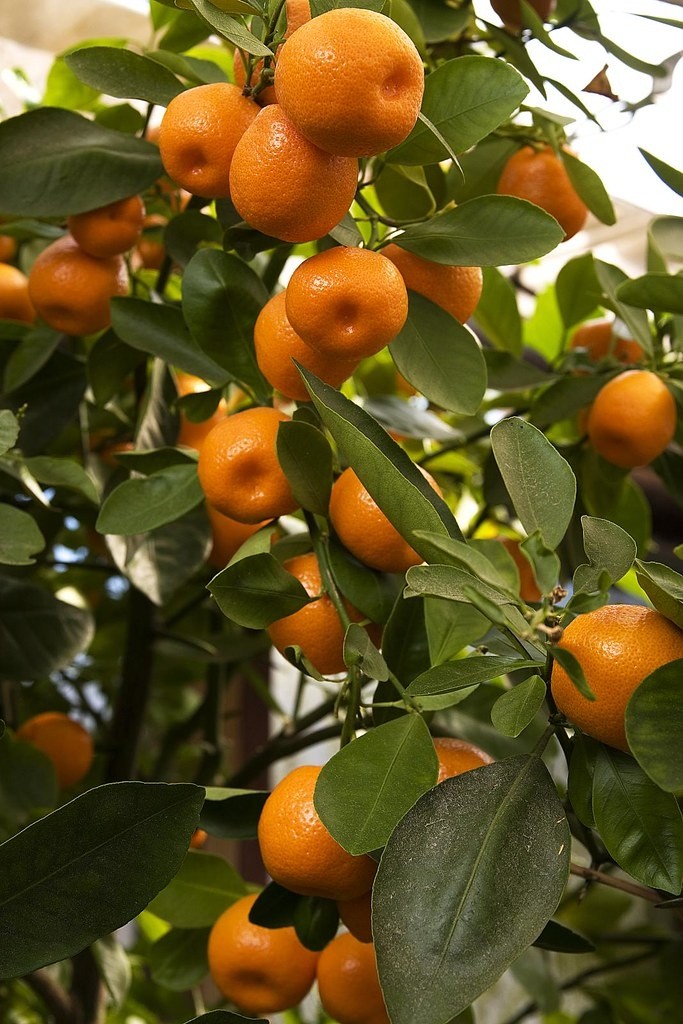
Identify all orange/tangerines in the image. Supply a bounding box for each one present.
[0,0,683,1024]
